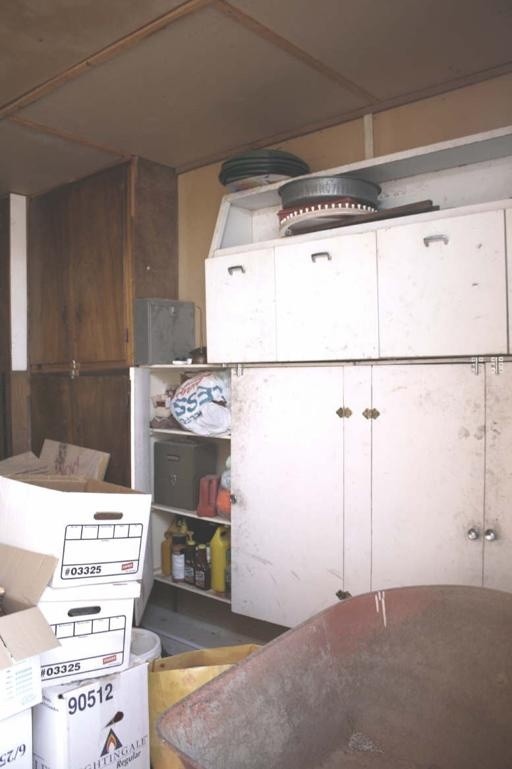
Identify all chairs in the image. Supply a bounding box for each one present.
[156,586,511,769]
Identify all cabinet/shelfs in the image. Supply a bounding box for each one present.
[130,365,236,607]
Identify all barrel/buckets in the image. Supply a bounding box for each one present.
[130,624,164,665]
[198,474,219,517]
[209,525,231,594]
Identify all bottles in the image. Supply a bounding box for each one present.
[160,517,210,592]
